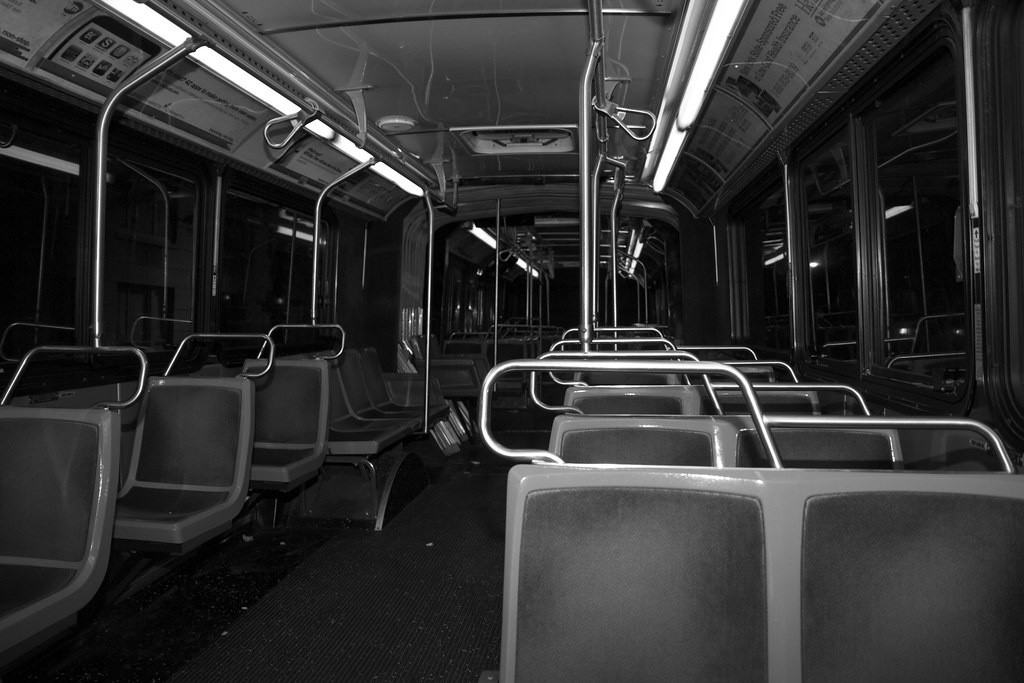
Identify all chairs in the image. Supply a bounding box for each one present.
[0,319,1024,683]
